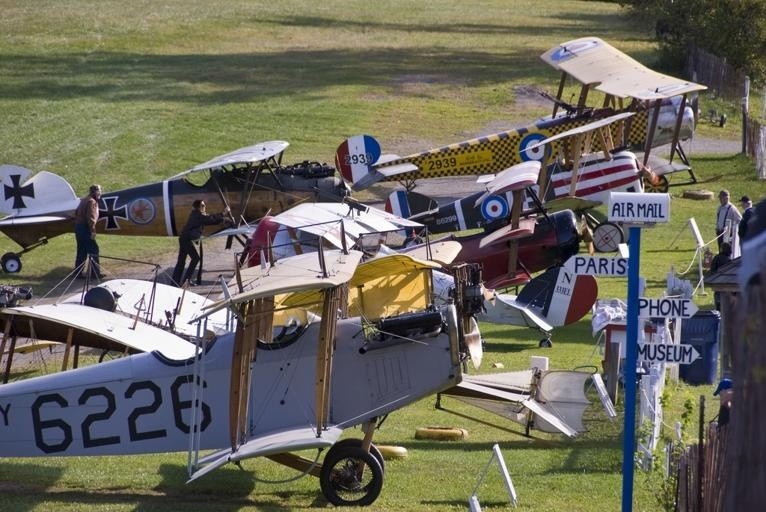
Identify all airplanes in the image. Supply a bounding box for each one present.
[333,34,708,194]
[1,110,727,508]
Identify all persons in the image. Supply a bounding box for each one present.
[712,378,734,428]
[171,199,231,286]
[738,195,753,248]
[70,184,109,280]
[715,190,744,260]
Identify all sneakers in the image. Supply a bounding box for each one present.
[183,277,197,286]
[72,272,107,279]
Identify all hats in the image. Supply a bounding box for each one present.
[739,195,750,202]
[713,379,733,396]
